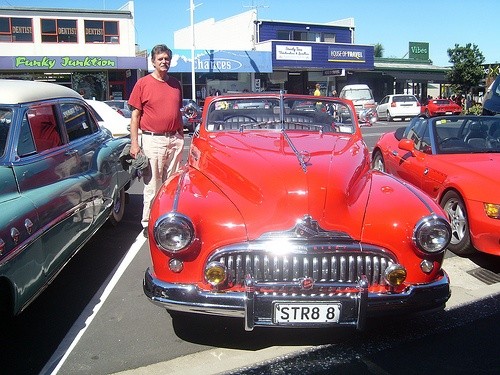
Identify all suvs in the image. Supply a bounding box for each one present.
[179,98,203,132]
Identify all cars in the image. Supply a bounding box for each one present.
[376,94,421,122]
[231,100,265,115]
[0,79,136,341]
[290,100,317,117]
[101,99,134,118]
[85,99,134,139]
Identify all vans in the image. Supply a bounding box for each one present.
[335,84,376,123]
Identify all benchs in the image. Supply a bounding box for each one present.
[224,114,330,131]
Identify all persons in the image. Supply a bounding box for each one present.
[467,91,472,110]
[127,44,184,238]
[110,94,114,100]
[209,86,221,96]
[314,84,322,111]
[328,90,338,116]
[214,101,229,130]
[417,94,465,107]
[29,114,104,233]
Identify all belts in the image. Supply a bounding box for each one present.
[142,128,180,136]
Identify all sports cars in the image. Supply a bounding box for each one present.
[421,99,462,118]
[372,110,500,258]
[142,89,453,331]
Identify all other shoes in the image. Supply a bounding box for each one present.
[143,226,148,238]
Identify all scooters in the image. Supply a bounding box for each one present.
[359,103,377,126]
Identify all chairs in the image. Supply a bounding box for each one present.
[489,122,500,136]
[464,124,489,143]
[420,122,441,145]
